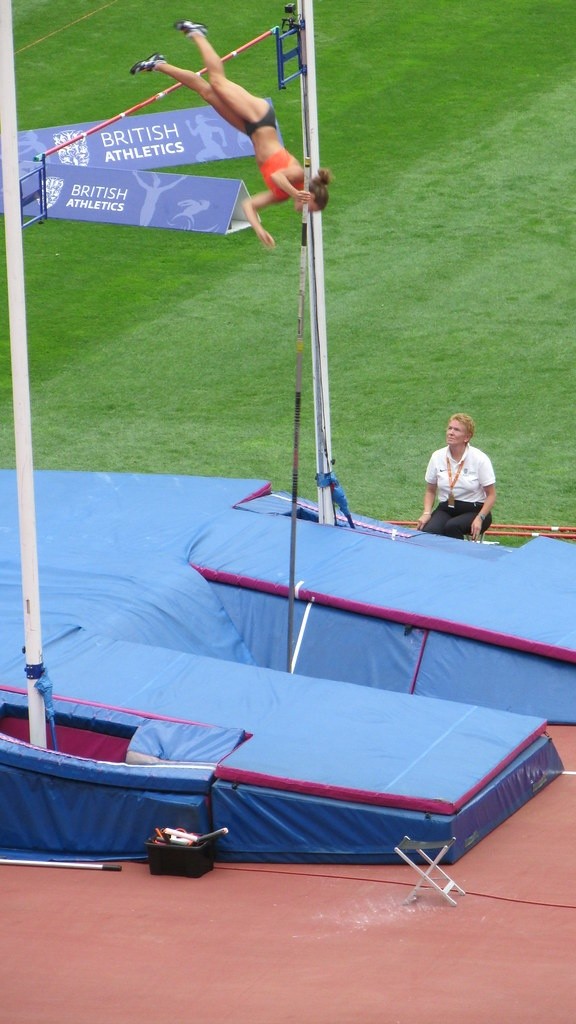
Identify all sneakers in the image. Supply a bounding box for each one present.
[174,21,207,38]
[130,52,166,75]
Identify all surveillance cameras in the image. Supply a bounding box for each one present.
[284,4,296,13]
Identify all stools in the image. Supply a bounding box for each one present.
[394,836,465,905]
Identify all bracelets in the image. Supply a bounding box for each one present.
[423,512,431,514]
[478,513,485,520]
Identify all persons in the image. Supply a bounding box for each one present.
[130,18,333,247]
[416,413,496,540]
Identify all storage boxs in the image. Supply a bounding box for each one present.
[145,834,215,877]
